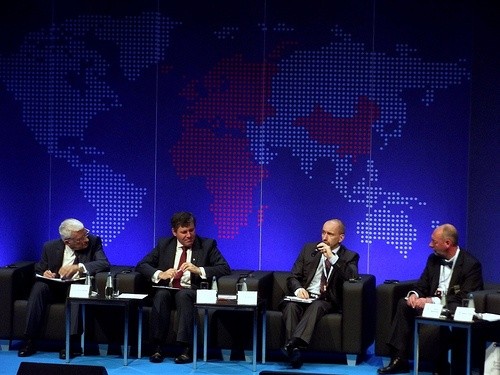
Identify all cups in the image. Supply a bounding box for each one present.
[200,282,209,289]
[111,278,119,298]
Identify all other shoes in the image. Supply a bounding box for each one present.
[290,351,304,369]
[281,343,293,356]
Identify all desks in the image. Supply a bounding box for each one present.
[413,315,492,375]
[191,299,268,369]
[65,290,147,366]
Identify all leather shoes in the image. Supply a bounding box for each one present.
[433,364,450,375]
[175,353,193,363]
[149,349,163,363]
[18,343,36,357]
[378,355,410,375]
[60,346,73,359]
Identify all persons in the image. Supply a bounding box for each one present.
[135,212,231,364]
[378,224,485,375]
[18,219,110,360]
[280,218,360,370]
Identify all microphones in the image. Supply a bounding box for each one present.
[311,246,323,257]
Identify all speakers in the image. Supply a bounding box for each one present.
[17,361,108,375]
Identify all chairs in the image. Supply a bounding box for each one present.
[0,260,500,375]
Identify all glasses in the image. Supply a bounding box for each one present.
[64,228,90,244]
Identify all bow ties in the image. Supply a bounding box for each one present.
[439,258,453,269]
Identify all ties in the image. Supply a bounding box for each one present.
[73,250,80,264]
[320,252,335,297]
[172,246,190,287]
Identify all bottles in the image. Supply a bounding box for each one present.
[468,293,475,308]
[104,270,114,298]
[442,294,448,315]
[85,272,92,294]
[242,278,247,291]
[211,276,218,301]
